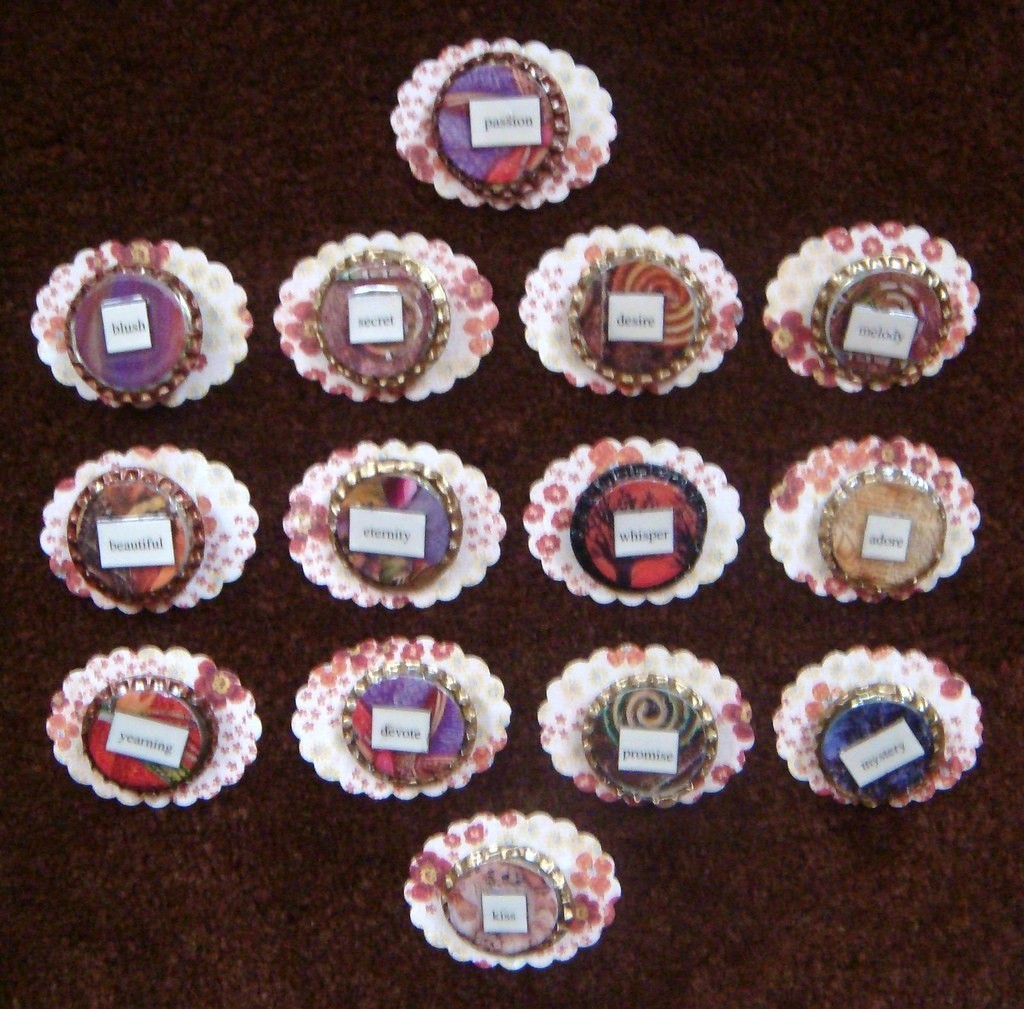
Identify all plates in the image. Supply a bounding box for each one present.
[519,223,743,395]
[403,811,620,971]
[538,642,754,805]
[274,231,499,403]
[31,236,253,408]
[773,643,982,808]
[522,435,745,606]
[47,644,262,809]
[390,36,616,208]
[290,636,512,801]
[283,436,507,610]
[762,221,980,392]
[762,435,981,603]
[39,444,258,614]
